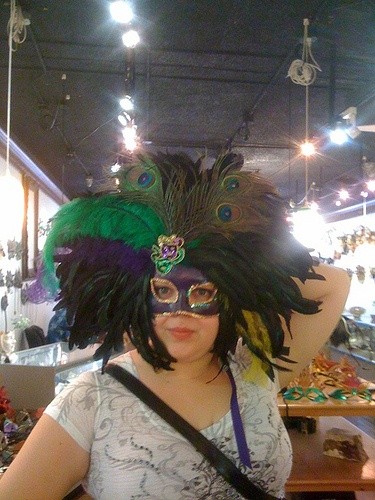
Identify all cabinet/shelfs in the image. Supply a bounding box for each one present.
[324,309,375,373]
[272,367,374,499]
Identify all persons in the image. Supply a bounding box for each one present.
[0,151,350,500]
